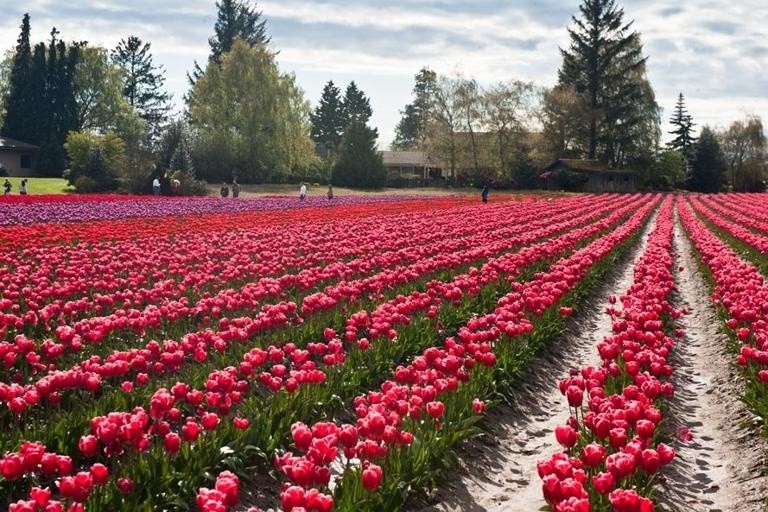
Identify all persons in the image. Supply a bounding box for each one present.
[300,181,307,199]
[220,182,229,197]
[170,175,180,195]
[481,185,488,203]
[232,179,240,197]
[327,184,333,199]
[153,176,161,195]
[3,178,27,195]
